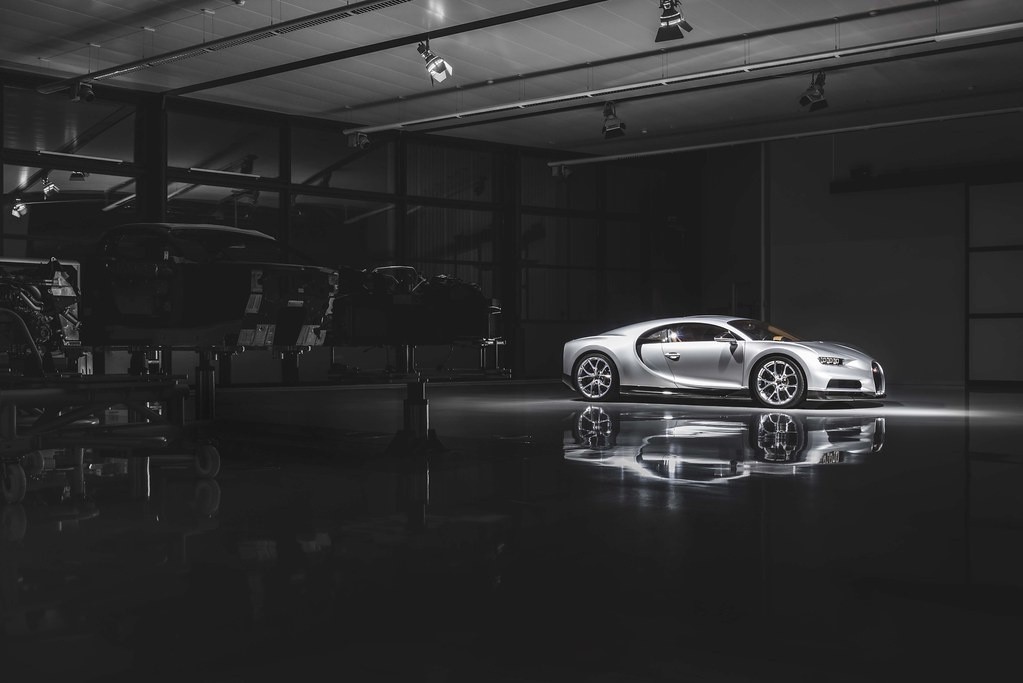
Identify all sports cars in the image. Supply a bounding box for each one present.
[559,394,887,488]
[560,314,888,408]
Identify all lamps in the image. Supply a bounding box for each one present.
[795,70,828,114]
[417,35,453,86]
[601,100,628,140]
[40,172,59,200]
[655,0,693,41]
